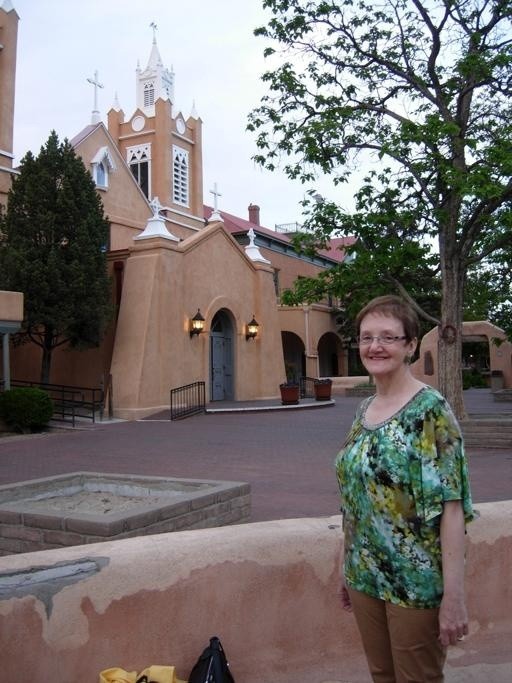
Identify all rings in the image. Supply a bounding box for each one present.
[457,634,466,641]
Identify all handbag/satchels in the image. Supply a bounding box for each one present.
[187,637,235,682]
[98,664,187,683]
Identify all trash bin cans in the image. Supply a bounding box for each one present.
[280,383,299,405]
[314,379,332,401]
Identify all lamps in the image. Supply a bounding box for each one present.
[246,314,259,341]
[189,306,205,337]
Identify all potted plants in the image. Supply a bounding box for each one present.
[279,378,332,404]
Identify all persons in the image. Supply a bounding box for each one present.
[332,291,477,682]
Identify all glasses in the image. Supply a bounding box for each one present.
[354,332,408,345]
